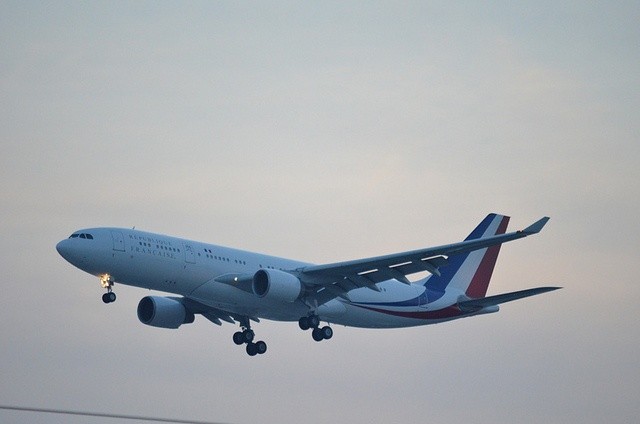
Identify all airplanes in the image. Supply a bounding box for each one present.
[56,213,563,356]
